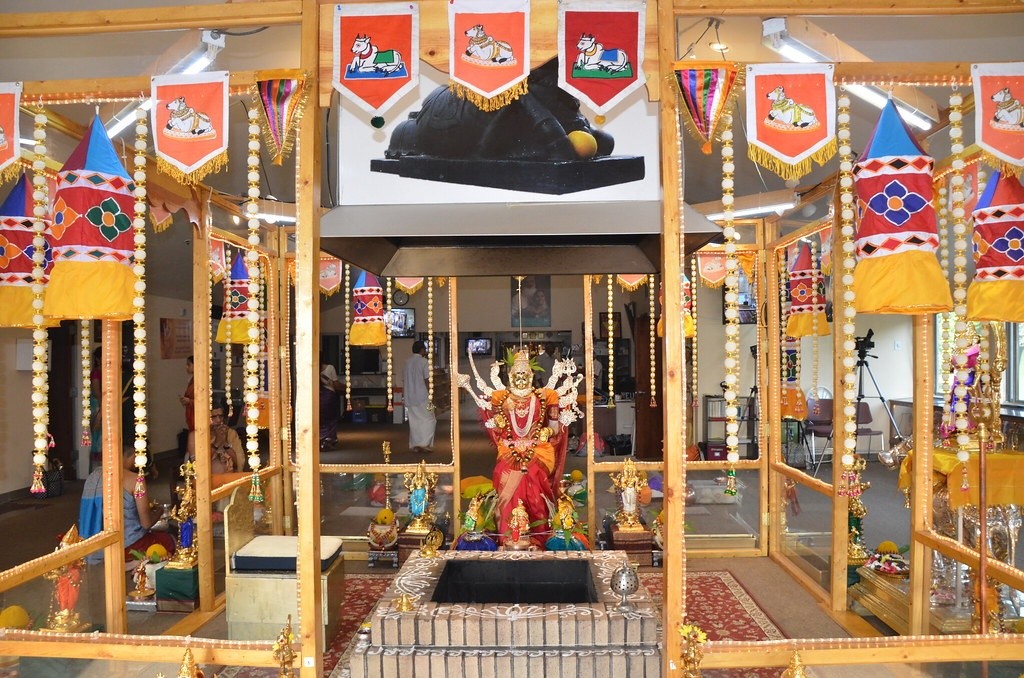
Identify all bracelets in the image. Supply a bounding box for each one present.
[211,443,218,450]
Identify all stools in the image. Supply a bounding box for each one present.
[351,409,366,424]
[364,405,386,426]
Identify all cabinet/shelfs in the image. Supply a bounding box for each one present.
[703,395,756,445]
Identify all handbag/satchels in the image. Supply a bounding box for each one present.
[606,433,632,455]
[33,451,64,499]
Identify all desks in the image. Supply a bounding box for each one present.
[339,386,394,424]
[889,398,1024,457]
[224,555,346,653]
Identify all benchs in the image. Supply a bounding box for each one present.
[800,399,884,469]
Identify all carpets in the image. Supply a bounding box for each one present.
[213,572,805,678]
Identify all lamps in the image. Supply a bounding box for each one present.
[102,27,272,141]
[763,18,942,129]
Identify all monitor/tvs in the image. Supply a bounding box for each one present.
[421,338,438,354]
[383,307,416,338]
[465,338,492,355]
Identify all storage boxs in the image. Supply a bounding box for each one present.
[156,565,198,616]
[610,446,631,457]
[781,421,798,446]
[707,444,727,461]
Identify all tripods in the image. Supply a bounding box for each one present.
[737,359,759,459]
[813,352,903,478]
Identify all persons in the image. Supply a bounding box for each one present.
[179,355,194,430]
[319,352,339,444]
[188,402,246,513]
[620,466,640,513]
[480,345,560,550]
[560,506,573,528]
[533,351,603,393]
[517,510,530,530]
[90,347,129,470]
[511,276,548,318]
[410,465,428,515]
[402,341,436,452]
[79,430,178,563]
[464,507,478,532]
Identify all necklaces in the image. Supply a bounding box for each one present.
[498,387,546,474]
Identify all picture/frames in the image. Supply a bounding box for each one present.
[510,275,551,327]
[598,311,623,340]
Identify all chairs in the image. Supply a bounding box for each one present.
[804,387,833,478]
[392,389,405,425]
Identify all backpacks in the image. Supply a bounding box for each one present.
[577,433,603,457]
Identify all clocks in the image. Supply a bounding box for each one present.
[392,289,409,306]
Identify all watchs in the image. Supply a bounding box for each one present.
[223,444,233,449]
